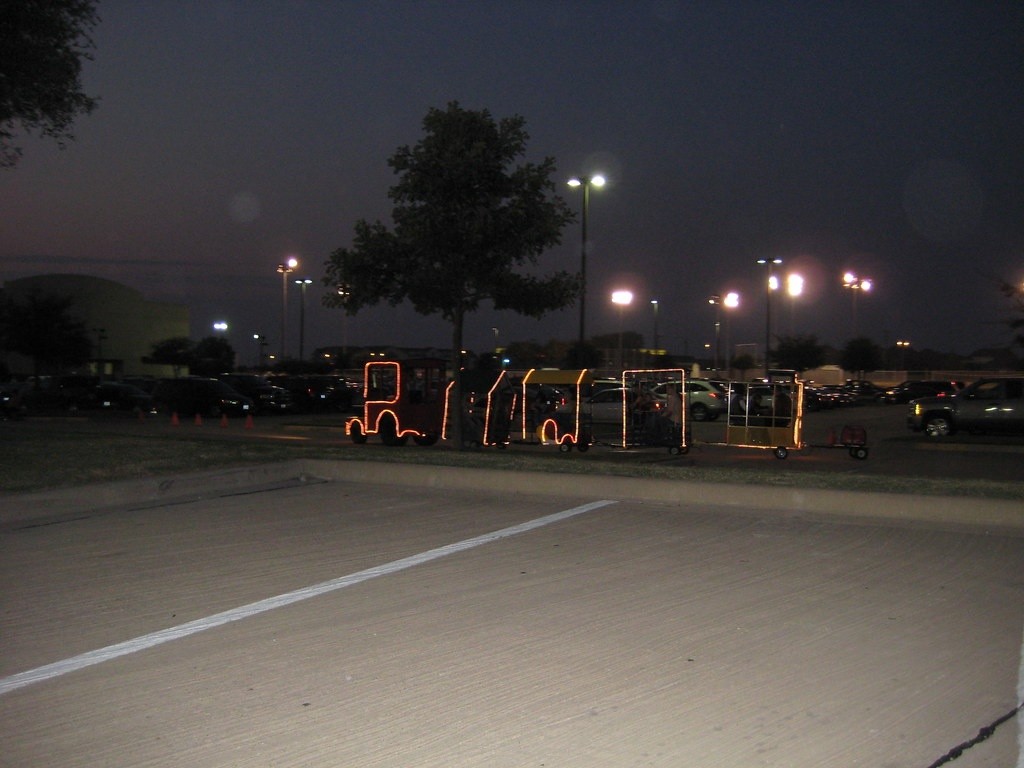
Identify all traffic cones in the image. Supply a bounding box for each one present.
[218,414,230,430]
[170,412,180,427]
[194,413,203,427]
[244,414,254,430]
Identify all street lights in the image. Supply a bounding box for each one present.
[609,287,636,353]
[651,299,659,348]
[295,279,314,360]
[842,272,872,342]
[276,256,298,361]
[722,291,740,369]
[755,257,784,379]
[565,171,607,344]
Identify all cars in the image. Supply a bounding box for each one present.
[805,379,963,411]
[556,387,668,427]
[0,369,366,423]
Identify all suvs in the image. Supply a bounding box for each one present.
[905,375,1024,443]
[651,380,725,422]
